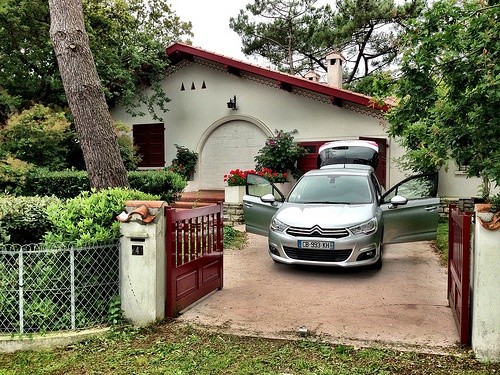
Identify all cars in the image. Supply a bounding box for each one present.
[242,139,441,272]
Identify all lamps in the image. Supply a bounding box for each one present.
[227,95,237,111]
